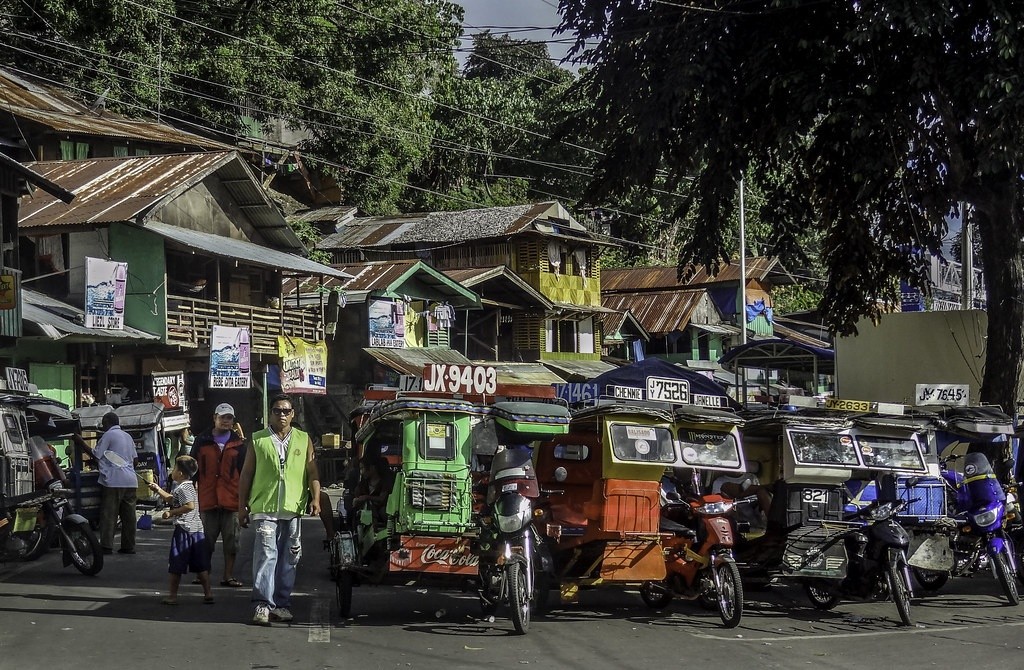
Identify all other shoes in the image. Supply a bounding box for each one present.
[99,546,113,553]
[203,595,215,604]
[117,549,136,554]
[161,600,177,604]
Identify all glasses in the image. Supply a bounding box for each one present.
[270,407,294,416]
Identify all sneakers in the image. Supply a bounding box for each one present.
[270,607,293,621]
[251,605,270,623]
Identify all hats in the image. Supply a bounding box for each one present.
[215,403,234,417]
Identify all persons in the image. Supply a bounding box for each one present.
[351,452,395,526]
[148,455,215,605]
[712,471,773,528]
[190,403,247,586]
[471,453,492,494]
[239,395,322,623]
[180,428,195,456]
[71,412,137,554]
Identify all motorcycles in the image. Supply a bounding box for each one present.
[0,366,105,577]
[320,365,572,633]
[845,380,1023,608]
[69,402,196,555]
[536,358,747,630]
[739,400,927,627]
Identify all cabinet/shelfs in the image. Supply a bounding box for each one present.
[80,366,118,408]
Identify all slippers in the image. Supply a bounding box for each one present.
[190,579,201,583]
[220,578,242,587]
